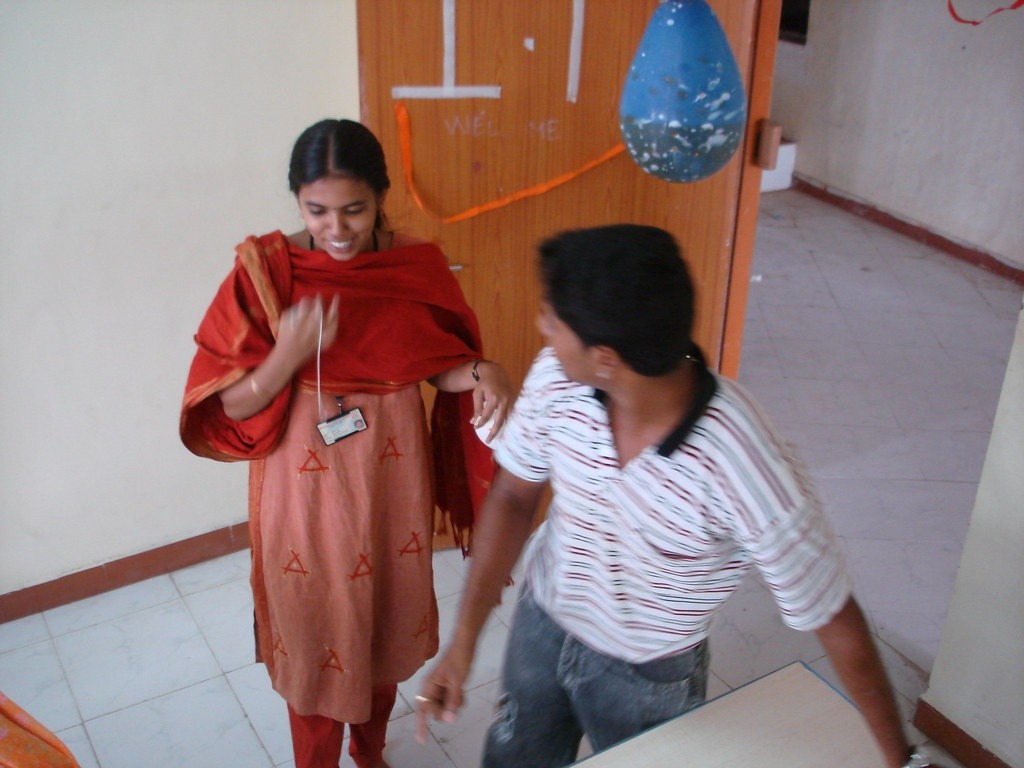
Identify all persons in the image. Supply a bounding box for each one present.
[354,420,363,428]
[180,119,513,768]
[413,224,950,768]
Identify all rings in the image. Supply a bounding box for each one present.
[417,695,432,702]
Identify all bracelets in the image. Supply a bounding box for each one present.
[901,753,930,768]
[472,359,493,382]
[250,375,270,404]
